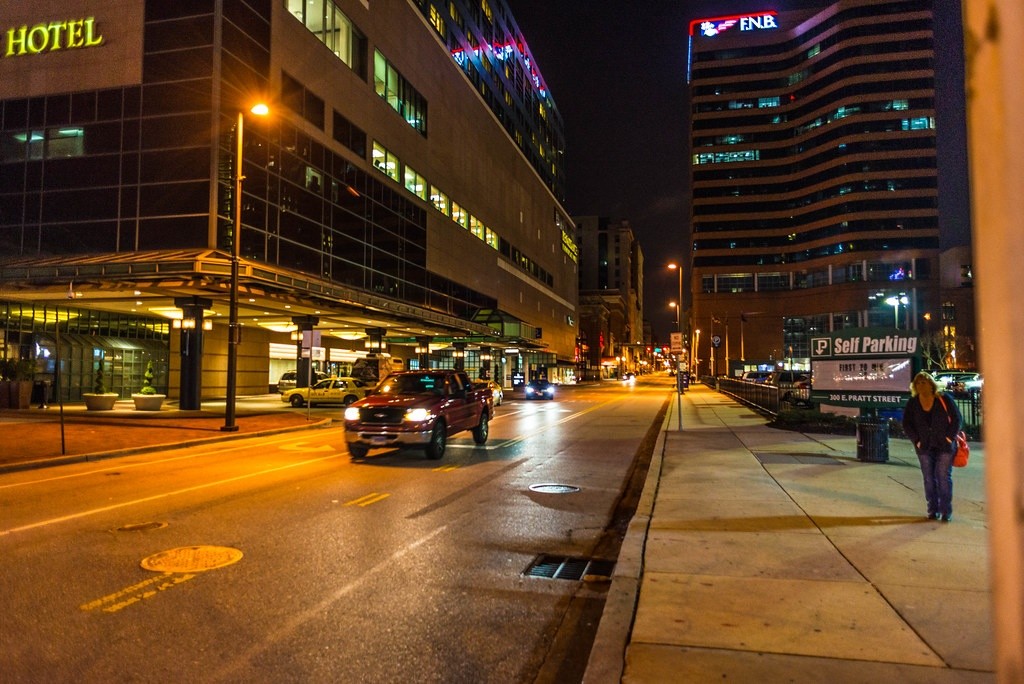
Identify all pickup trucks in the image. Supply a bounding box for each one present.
[343,370,493,462]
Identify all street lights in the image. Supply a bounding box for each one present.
[220,97,269,433]
[667,263,684,432]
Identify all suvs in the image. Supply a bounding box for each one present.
[790,373,814,409]
[763,370,810,402]
[277,370,329,396]
[743,370,772,385]
[282,377,378,409]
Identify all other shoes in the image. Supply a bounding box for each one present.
[929,512,940,520]
[942,512,952,521]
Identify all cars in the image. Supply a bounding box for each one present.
[623,373,636,381]
[526,379,555,400]
[472,379,505,405]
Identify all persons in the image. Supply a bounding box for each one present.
[903,372,962,522]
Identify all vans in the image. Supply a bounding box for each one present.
[932,371,983,399]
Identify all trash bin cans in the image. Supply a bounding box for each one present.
[783,398,813,412]
[856,417,890,462]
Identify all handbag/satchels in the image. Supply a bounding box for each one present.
[953,431,969,466]
